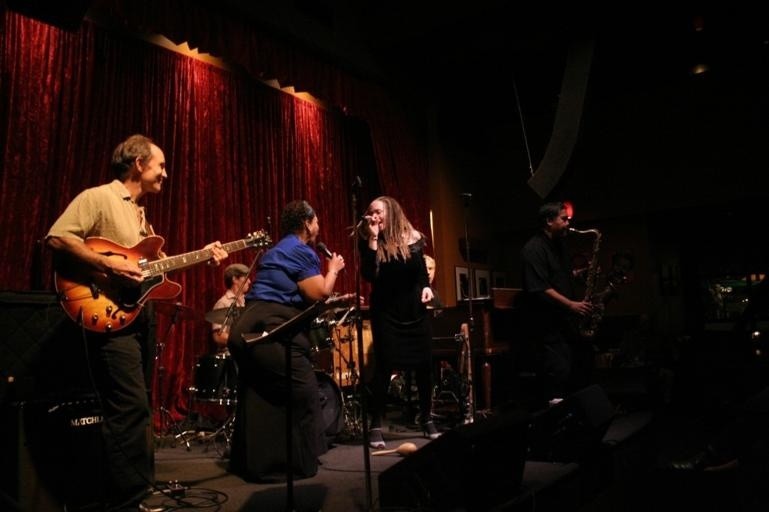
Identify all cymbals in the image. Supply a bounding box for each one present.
[204,307,247,325]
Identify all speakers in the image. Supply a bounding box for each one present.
[377,416,529,512]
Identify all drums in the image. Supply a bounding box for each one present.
[191,398,238,421]
[308,325,332,354]
[314,370,343,428]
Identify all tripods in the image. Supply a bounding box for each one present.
[151,314,192,452]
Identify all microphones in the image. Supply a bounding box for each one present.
[317,242,347,274]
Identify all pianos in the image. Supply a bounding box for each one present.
[432,288,522,410]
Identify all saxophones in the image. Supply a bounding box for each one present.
[568,227,618,338]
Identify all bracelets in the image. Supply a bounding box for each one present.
[369,233,379,241]
[328,269,338,277]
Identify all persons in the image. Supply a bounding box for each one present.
[42,135,232,512]
[350,194,443,451]
[421,254,443,308]
[605,251,649,368]
[224,194,367,483]
[506,198,596,463]
[207,263,253,350]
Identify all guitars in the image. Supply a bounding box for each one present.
[49,230,272,333]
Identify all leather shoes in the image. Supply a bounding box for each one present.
[424,420,442,441]
[369,428,388,450]
